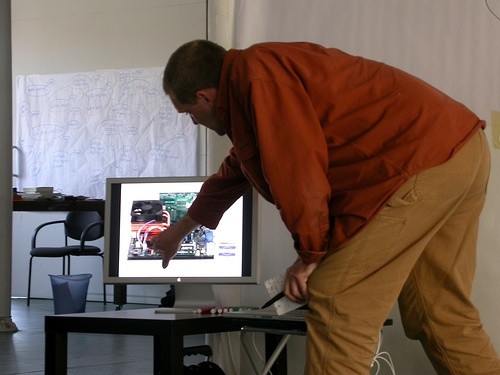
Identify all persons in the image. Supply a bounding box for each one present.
[143,39,500,375]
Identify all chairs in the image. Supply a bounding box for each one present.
[25,201,108,309]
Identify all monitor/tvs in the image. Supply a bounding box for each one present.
[104,176,261,284]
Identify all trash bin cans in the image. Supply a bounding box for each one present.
[47,271,93,314]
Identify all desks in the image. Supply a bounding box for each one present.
[41,306,393,375]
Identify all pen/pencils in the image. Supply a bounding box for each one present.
[196,304,258,314]
[260,291,285,309]
